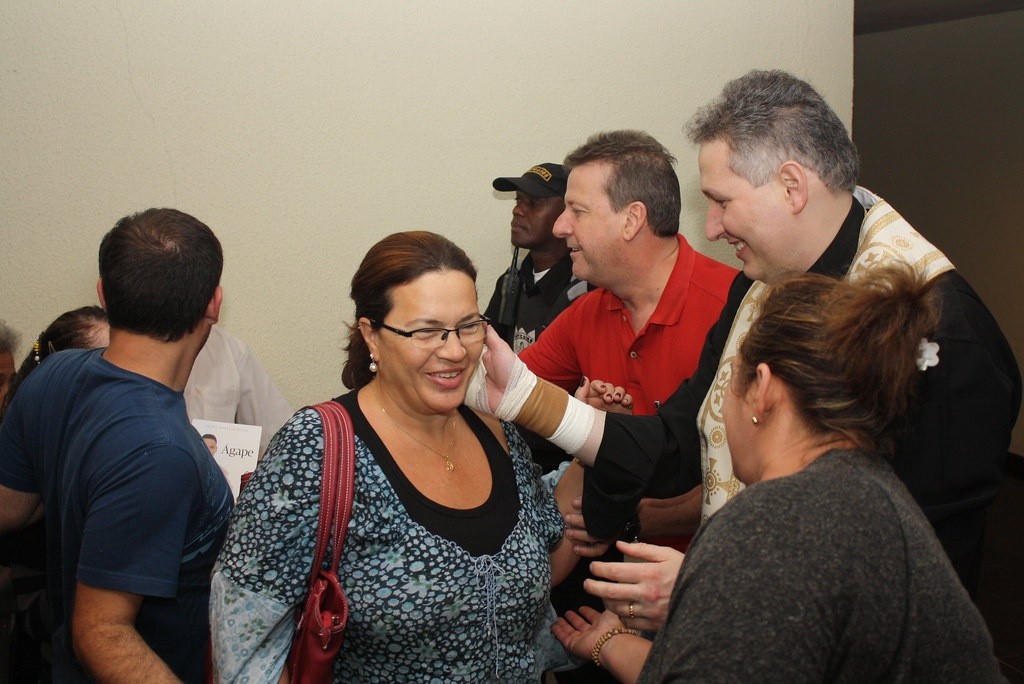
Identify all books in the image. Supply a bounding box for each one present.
[190,419,263,504]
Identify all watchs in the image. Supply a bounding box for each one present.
[620,514,641,543]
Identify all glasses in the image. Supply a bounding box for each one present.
[358,314,490,350]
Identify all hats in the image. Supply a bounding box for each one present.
[493,162,570,199]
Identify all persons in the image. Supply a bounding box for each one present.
[0,207,235,684]
[184,324,295,461]
[516,128,741,557]
[463,68,1024,684]
[551,272,1010,684]
[209,230,566,684]
[202,434,218,457]
[483,163,598,476]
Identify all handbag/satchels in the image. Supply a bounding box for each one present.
[277,400,356,684]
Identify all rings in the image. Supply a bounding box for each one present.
[629,602,635,619]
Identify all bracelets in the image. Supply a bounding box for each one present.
[592,626,637,666]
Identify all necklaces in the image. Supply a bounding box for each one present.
[371,379,457,472]
